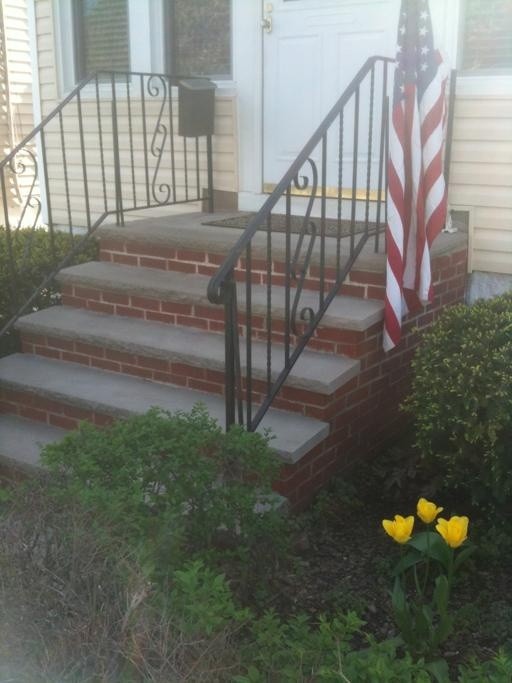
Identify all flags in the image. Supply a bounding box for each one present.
[383,0,449,353]
